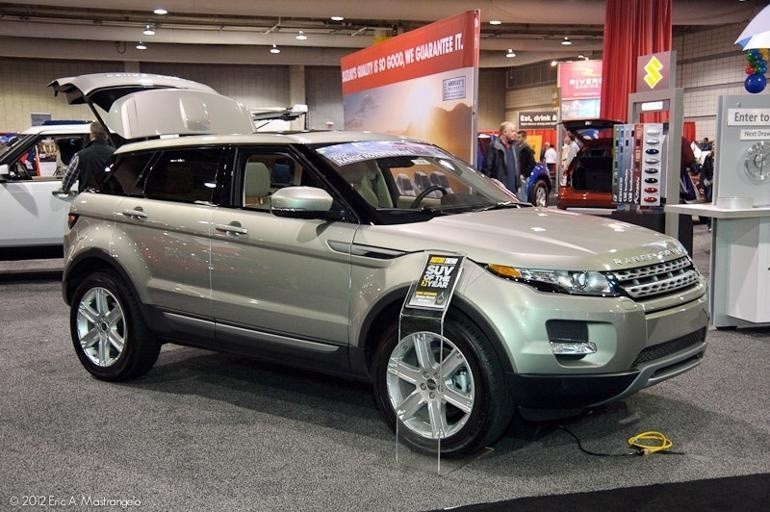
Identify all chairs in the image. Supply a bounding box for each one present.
[245,162,275,211]
[330,162,378,210]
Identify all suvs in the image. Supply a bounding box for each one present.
[45,73,714,460]
[0,103,310,262]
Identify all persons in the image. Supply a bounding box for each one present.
[514,130,536,202]
[681,135,715,232]
[481,120,522,201]
[540,130,581,176]
[59,121,118,194]
